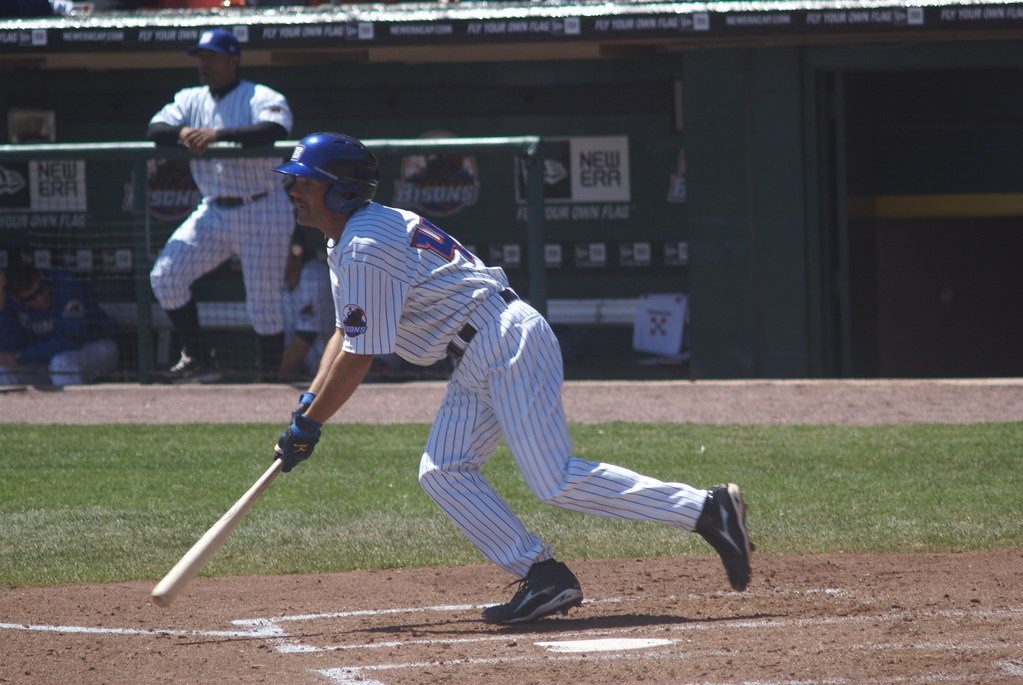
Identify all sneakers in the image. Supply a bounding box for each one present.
[146,345,224,382]
[256,358,284,384]
[482,557,585,624]
[694,482,754,593]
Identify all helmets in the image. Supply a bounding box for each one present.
[270,131,379,212]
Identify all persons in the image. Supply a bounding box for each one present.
[277,233,336,380]
[147,29,293,383]
[270,132,755,622]
[0,261,117,388]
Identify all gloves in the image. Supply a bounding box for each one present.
[289,392,316,427]
[274,413,323,473]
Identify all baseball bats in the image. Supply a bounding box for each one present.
[151,456,285,609]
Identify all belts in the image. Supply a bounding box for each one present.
[445,289,518,359]
[214,192,268,208]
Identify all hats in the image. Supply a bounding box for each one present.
[187,29,241,59]
